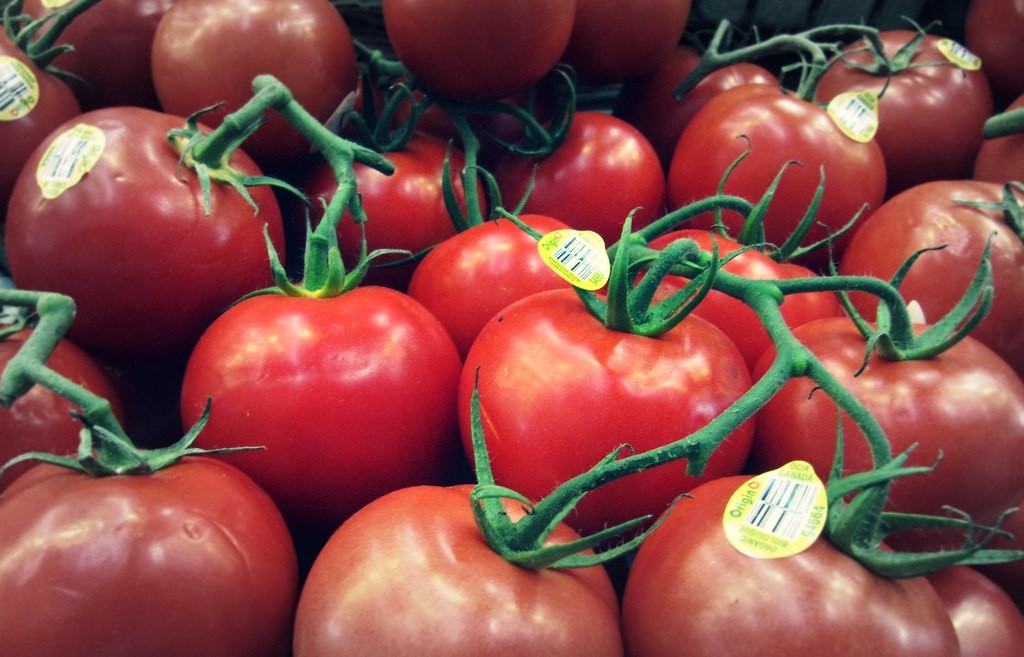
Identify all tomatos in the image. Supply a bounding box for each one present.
[0,0,1024,657]
